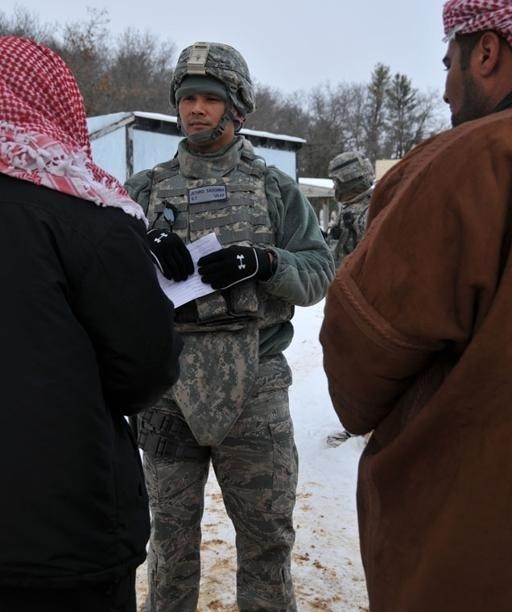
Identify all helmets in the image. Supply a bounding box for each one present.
[168,41,255,114]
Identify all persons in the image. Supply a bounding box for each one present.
[0,35,185,611]
[320,151,376,269]
[320,0,512,612]
[123,42,335,612]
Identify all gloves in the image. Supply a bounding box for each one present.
[197,244,278,290]
[143,228,195,282]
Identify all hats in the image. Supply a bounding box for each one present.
[328,151,374,184]
